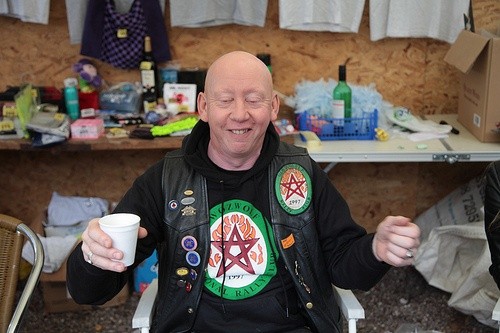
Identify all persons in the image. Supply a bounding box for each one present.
[67,51,421,333]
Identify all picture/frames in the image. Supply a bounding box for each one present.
[163,84,196,113]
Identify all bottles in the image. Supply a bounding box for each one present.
[140,36,158,100]
[257,52,271,74]
[332,65,352,125]
[64,81,79,120]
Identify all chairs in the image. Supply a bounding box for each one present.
[131,276,365,333]
[0,214,45,333]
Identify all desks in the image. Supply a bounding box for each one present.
[0,109,199,150]
[293,114,500,174]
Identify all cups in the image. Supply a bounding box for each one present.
[98,213,140,267]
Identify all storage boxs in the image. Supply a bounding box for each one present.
[19,201,127,313]
[444,28,500,143]
[70,119,105,140]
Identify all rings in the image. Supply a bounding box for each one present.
[406,248,415,260]
[87,252,94,264]
